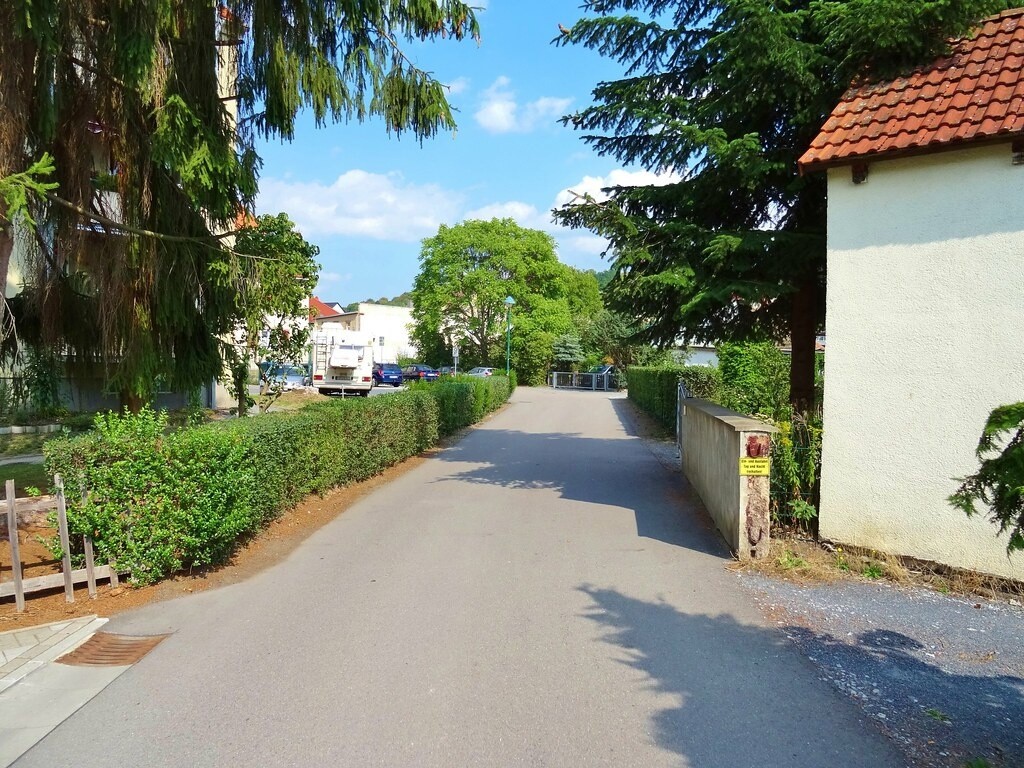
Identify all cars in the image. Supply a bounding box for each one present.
[400,364,440,383]
[465,367,497,377]
[371,363,403,387]
[581,365,616,388]
[261,363,312,394]
[436,367,463,377]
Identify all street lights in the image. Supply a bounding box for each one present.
[503,296,516,375]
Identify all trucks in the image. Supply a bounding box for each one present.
[312,322,374,397]
